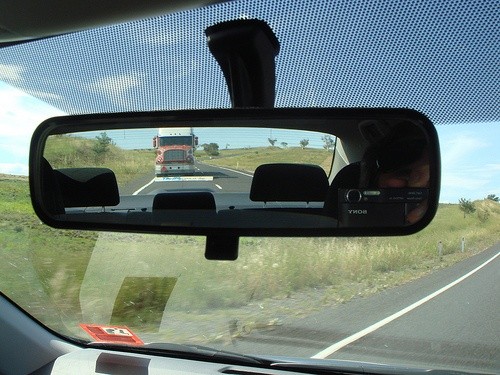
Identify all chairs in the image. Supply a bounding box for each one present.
[238,163,330,229]
[53,168,128,223]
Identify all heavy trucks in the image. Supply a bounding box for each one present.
[153,128,199,177]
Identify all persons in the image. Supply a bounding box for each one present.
[321,119,430,227]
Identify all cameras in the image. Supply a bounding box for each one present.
[337,187,430,229]
